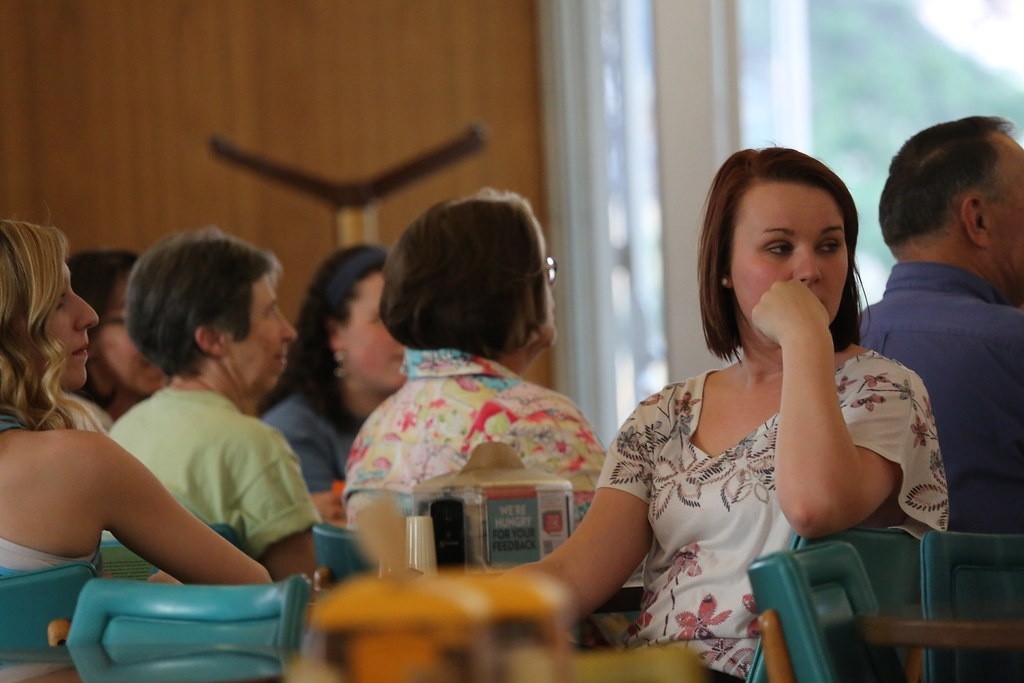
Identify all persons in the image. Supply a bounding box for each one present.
[64,191,648,654]
[480,146,950,683]
[0,217,274,587]
[856,116,1023,536]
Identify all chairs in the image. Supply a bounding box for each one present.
[100,524,242,583]
[920,529,1024,683]
[47,576,311,651]
[746,527,912,683]
[0,560,98,649]
[313,522,379,598]
[746,542,904,683]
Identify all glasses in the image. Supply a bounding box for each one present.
[514,256,558,285]
